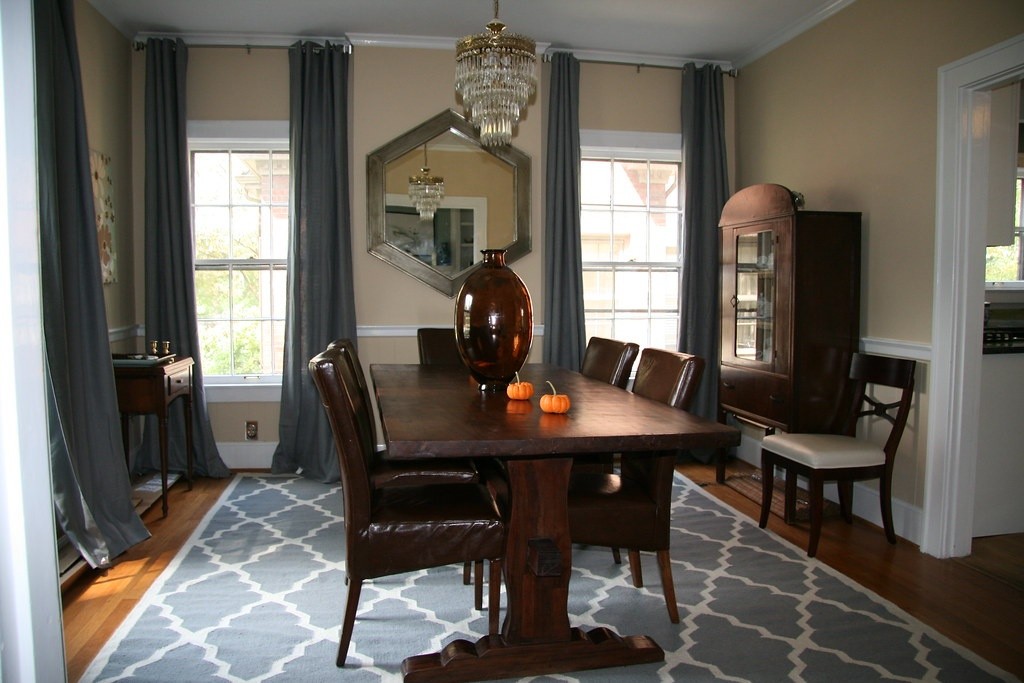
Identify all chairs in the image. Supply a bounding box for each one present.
[567,349,706,624]
[307,328,506,667]
[576,337,640,563]
[755,354,916,556]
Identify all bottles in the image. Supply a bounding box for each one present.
[454,250,533,392]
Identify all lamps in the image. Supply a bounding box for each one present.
[453,0,537,147]
[409,143,445,222]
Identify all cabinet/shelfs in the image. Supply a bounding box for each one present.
[450,209,474,273]
[717,183,861,525]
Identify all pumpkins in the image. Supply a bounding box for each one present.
[507,371,534,400]
[539,413,569,428]
[506,399,532,414]
[539,381,570,413]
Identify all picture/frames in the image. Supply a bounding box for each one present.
[386,210,436,256]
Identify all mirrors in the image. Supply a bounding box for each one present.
[365,108,533,298]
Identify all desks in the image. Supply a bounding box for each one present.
[108,356,195,517]
[370,363,742,683]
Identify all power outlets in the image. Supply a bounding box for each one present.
[245,421,258,440]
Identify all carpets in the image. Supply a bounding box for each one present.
[77,468,1021,683]
